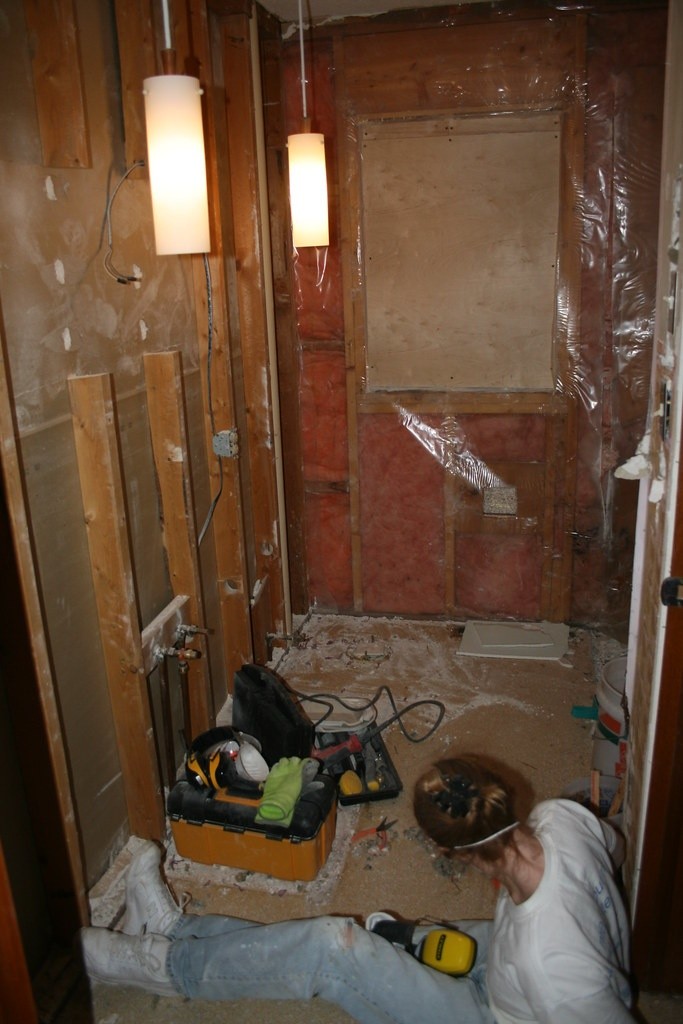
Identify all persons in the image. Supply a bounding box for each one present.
[82,760,637,1024]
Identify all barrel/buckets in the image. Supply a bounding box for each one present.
[591,656,627,778]
[561,775,625,831]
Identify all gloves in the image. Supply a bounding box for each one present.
[254,757,324,826]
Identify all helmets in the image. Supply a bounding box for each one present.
[184,725,245,791]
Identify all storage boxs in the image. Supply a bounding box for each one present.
[168,764,337,880]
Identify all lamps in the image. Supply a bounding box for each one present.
[140,0,213,260]
[285,0,330,249]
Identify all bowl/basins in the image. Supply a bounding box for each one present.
[235,746,269,781]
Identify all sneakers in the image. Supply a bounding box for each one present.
[70,926,182,996]
[123,841,191,936]
